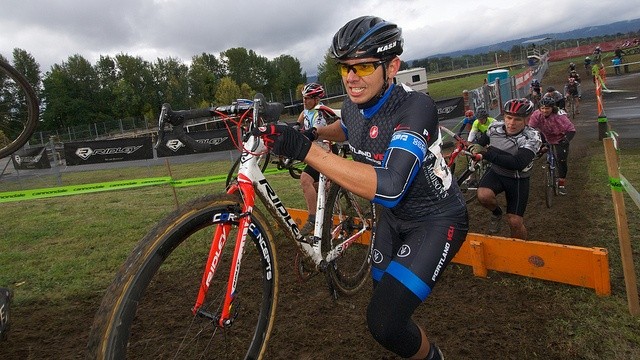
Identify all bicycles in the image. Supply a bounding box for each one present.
[84,92,379,360]
[563,96,581,120]
[439,126,493,205]
[535,142,560,208]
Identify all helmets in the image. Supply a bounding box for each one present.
[570,63,573,65]
[596,46,599,49]
[477,110,488,119]
[465,110,474,117]
[302,83,325,98]
[504,98,535,117]
[329,16,404,60]
[532,79,538,83]
[538,97,555,107]
[569,78,575,83]
[547,87,555,92]
[585,57,589,59]
[571,70,576,73]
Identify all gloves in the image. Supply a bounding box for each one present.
[467,144,488,154]
[253,125,317,162]
[561,138,569,146]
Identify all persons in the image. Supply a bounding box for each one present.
[525,80,565,110]
[293,83,341,236]
[565,78,582,114]
[466,98,543,240]
[482,78,492,104]
[528,97,576,196]
[594,46,601,59]
[622,40,640,47]
[467,109,497,146]
[584,57,592,70]
[566,63,581,87]
[612,54,622,76]
[615,46,622,58]
[252,16,469,360]
[456,110,477,141]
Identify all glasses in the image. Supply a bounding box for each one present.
[335,59,388,78]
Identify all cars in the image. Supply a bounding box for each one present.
[41,141,66,162]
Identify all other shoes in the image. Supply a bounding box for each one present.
[489,214,502,233]
[575,110,580,114]
[431,343,445,360]
[566,103,570,108]
[558,178,568,195]
[300,219,315,236]
